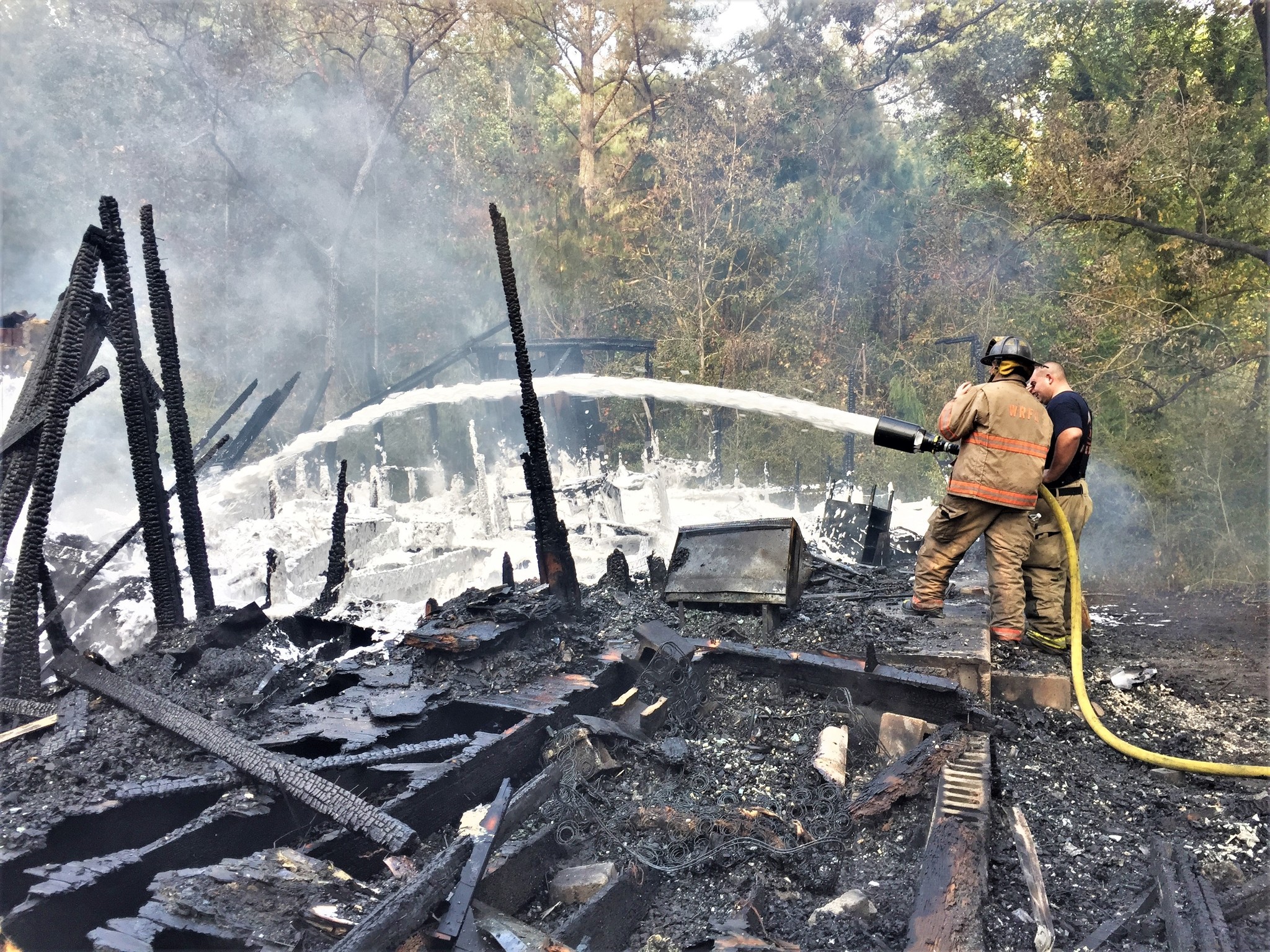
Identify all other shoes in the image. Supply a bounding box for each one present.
[900,599,942,618]
[1018,635,1061,656]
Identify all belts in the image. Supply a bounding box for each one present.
[1037,486,1085,498]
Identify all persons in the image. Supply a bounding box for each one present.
[1026,361,1093,654]
[901,336,1054,649]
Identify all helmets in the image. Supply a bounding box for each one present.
[980,337,1047,369]
